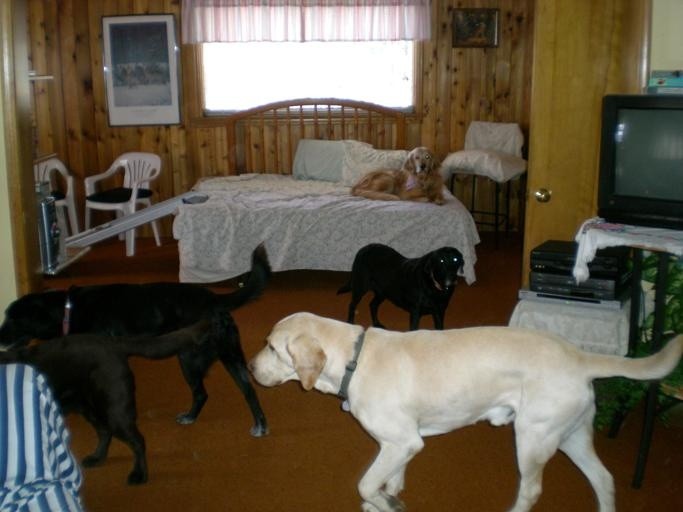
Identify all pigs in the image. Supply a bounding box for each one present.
[0,239,272,438]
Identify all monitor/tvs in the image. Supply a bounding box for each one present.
[597,94,683,231]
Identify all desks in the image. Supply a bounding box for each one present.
[573,217,683,489]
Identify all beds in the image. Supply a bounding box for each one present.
[174,98,480,286]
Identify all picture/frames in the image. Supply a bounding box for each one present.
[451,9,500,47]
[102,14,179,124]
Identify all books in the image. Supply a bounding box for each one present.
[643,69,683,95]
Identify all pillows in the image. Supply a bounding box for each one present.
[291,139,372,186]
[86,187,153,203]
[438,150,528,184]
[342,142,412,192]
[50,190,65,200]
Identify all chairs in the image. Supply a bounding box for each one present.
[32,159,79,238]
[439,120,527,247]
[85,154,162,260]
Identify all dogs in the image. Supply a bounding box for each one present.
[335,242,464,334]
[245,310,682,511]
[350,148,447,206]
[0,332,194,487]
